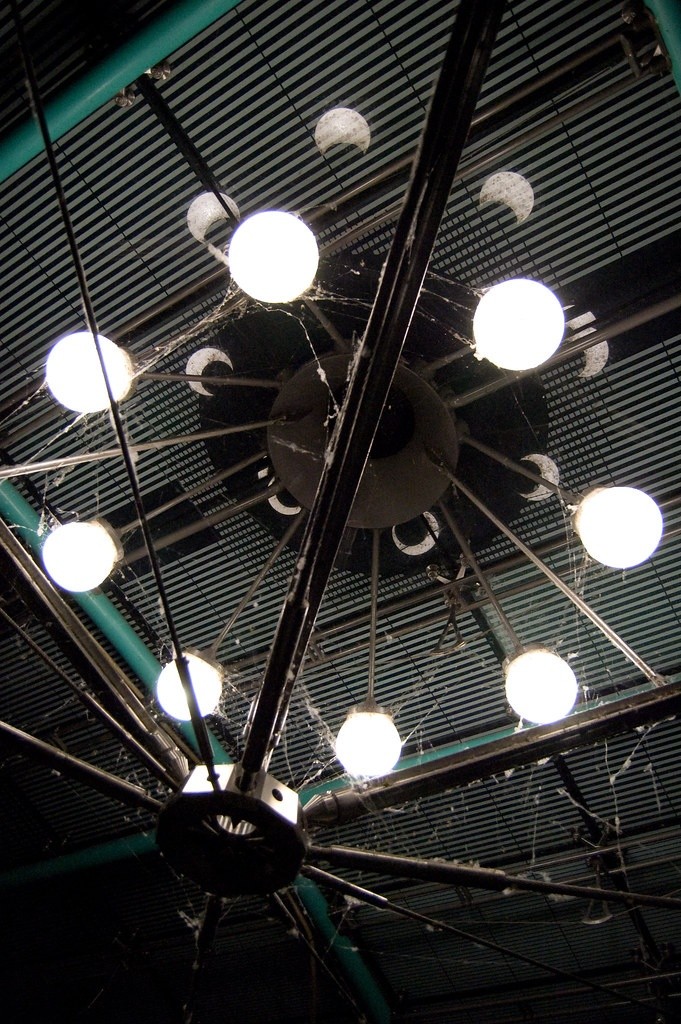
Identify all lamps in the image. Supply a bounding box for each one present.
[156,507,304,718]
[186,346,234,395]
[478,169,535,232]
[45,330,281,414]
[566,326,609,380]
[417,278,565,377]
[268,474,304,515]
[460,433,659,569]
[506,451,561,502]
[227,211,349,354]
[315,107,371,169]
[41,450,267,593]
[392,512,440,555]
[336,529,402,778]
[186,192,241,249]
[436,498,578,722]
